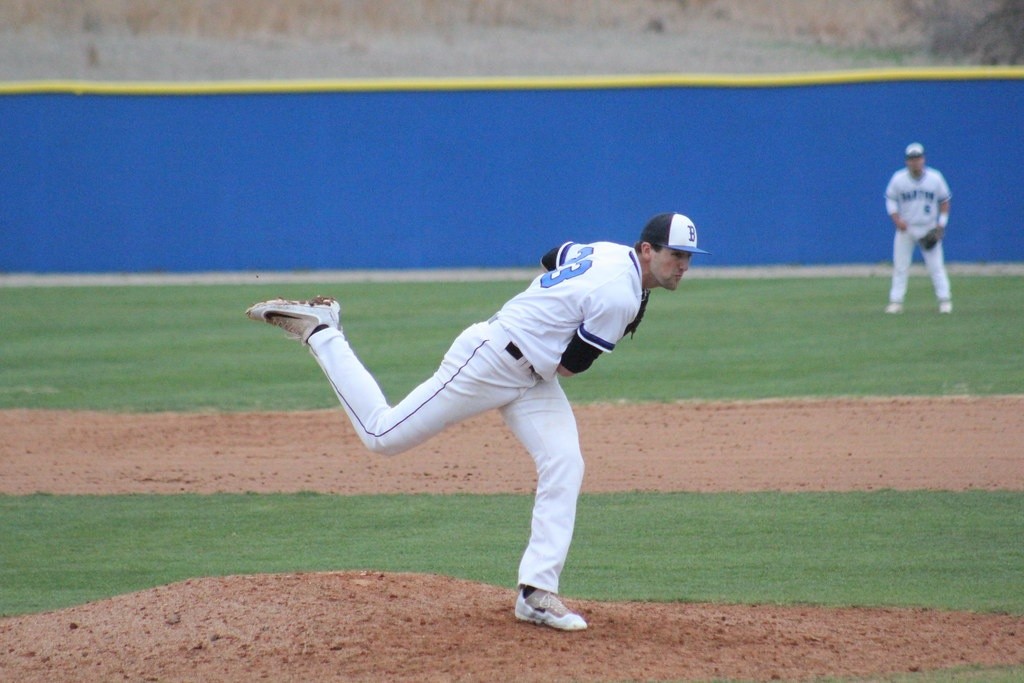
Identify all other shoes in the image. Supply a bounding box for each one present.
[884,303,904,315]
[939,300,954,314]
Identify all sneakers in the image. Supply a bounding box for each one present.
[513,585,589,632]
[245,295,341,346]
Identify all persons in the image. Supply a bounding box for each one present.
[243,213,713,632]
[885,141,956,314]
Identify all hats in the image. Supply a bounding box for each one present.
[904,141,926,157]
[639,211,710,255]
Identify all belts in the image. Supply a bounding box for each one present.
[506,341,536,374]
